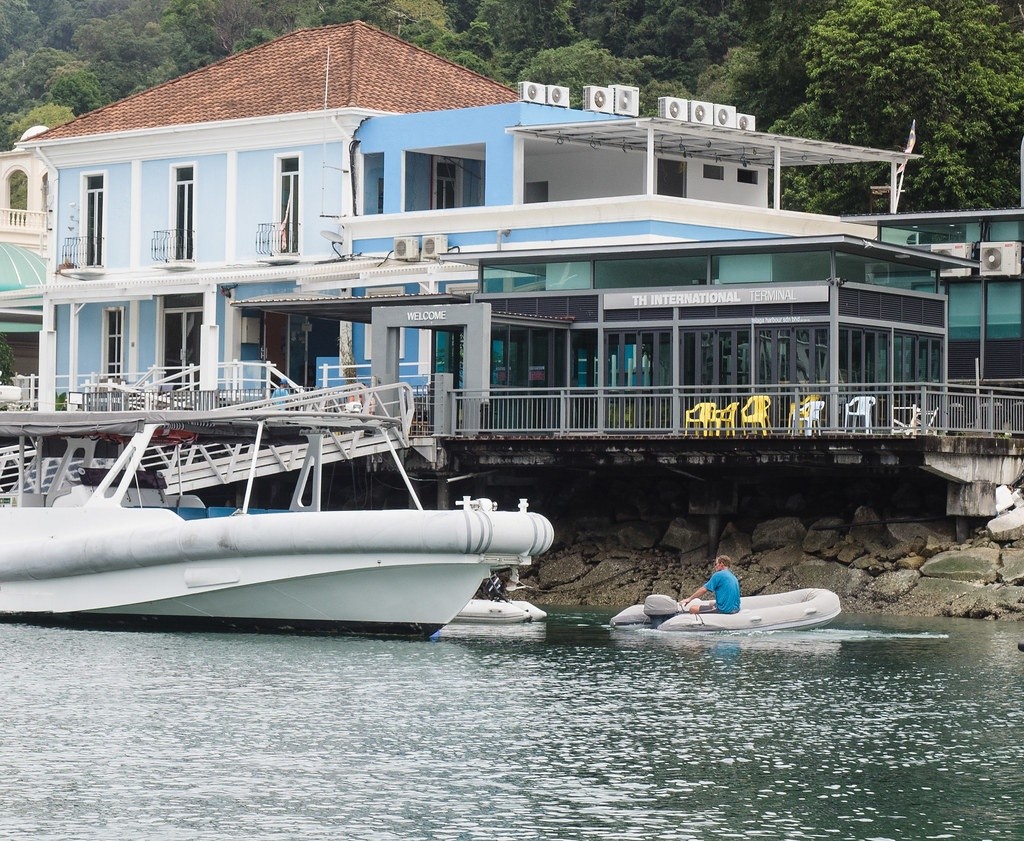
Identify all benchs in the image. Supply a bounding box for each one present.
[127,505,290,522]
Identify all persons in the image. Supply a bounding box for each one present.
[267,377,294,411]
[679,553,740,614]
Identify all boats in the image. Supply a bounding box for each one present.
[453,599,549,625]
[610,586,842,637]
[0,384,554,644]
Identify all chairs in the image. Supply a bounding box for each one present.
[791,401,825,436]
[741,395,774,436]
[893,404,939,433]
[787,393,821,435]
[685,402,718,437]
[843,395,876,434]
[706,401,740,437]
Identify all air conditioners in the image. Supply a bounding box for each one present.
[930,241,975,279]
[422,235,451,257]
[517,82,756,137]
[394,236,419,258]
[978,241,1024,276]
